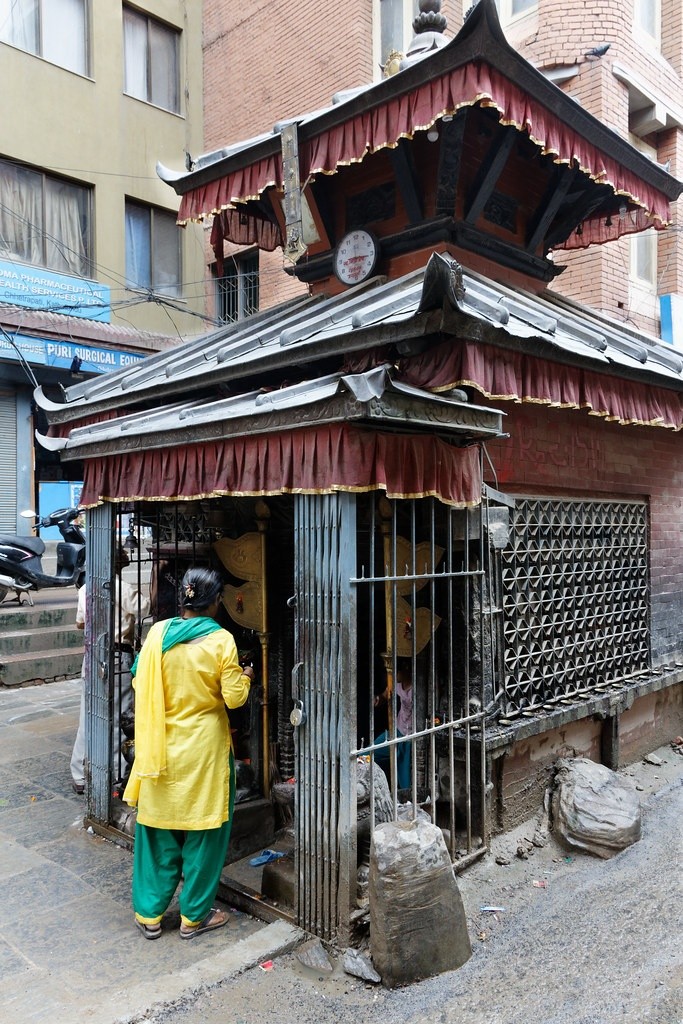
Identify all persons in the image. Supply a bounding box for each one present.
[122,568,254,940]
[70,574,152,794]
[374,657,413,789]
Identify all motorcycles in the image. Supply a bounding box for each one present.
[0,507,89,607]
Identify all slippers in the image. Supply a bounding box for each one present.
[249,849,285,866]
[179,907,230,939]
[133,916,163,940]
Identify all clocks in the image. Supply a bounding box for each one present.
[332,226,379,287]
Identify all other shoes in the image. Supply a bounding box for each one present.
[73,781,84,794]
